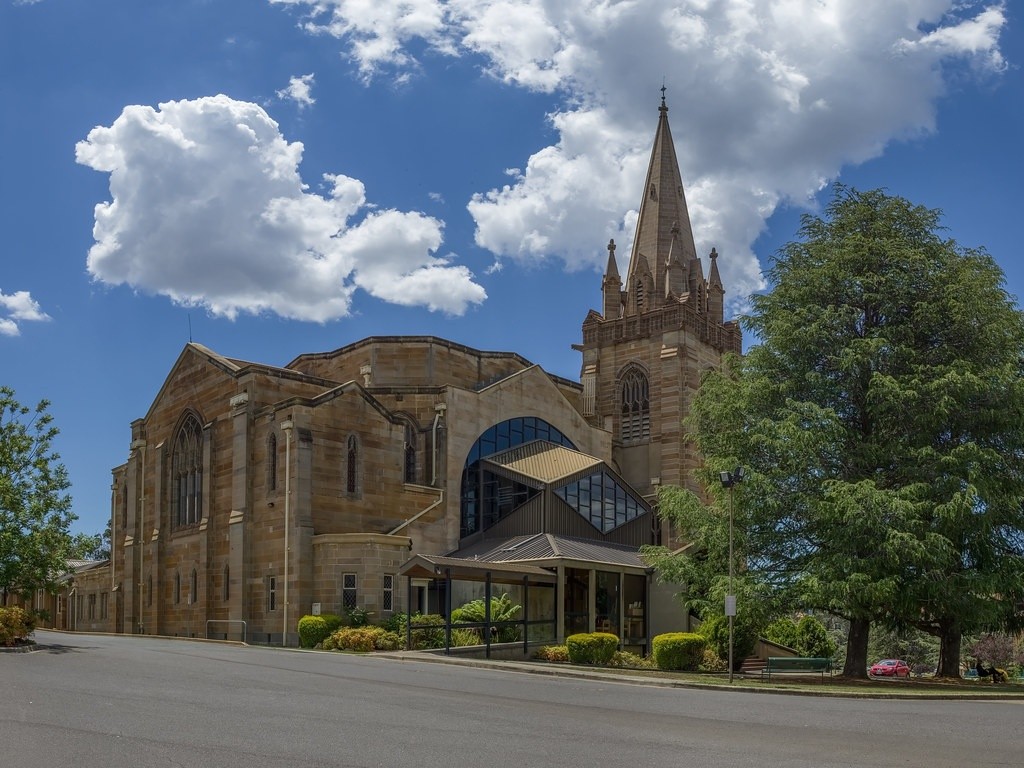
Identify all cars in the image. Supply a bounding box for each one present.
[869,659,912,679]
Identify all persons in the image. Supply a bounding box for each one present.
[976,660,1004,684]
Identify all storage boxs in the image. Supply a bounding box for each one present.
[627,607,643,616]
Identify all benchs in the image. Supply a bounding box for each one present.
[761,657,834,682]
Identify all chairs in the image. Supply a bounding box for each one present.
[624,618,631,638]
[596,619,610,633]
